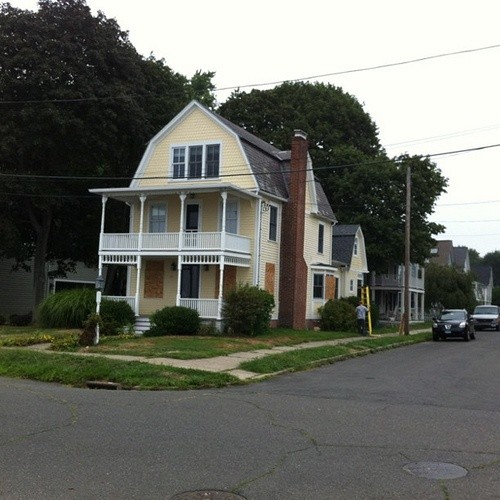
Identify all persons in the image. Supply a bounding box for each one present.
[354,301,371,337]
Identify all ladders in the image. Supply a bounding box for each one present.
[361,285,373,336]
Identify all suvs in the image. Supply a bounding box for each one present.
[469,305,500,331]
[431,308,477,341]
